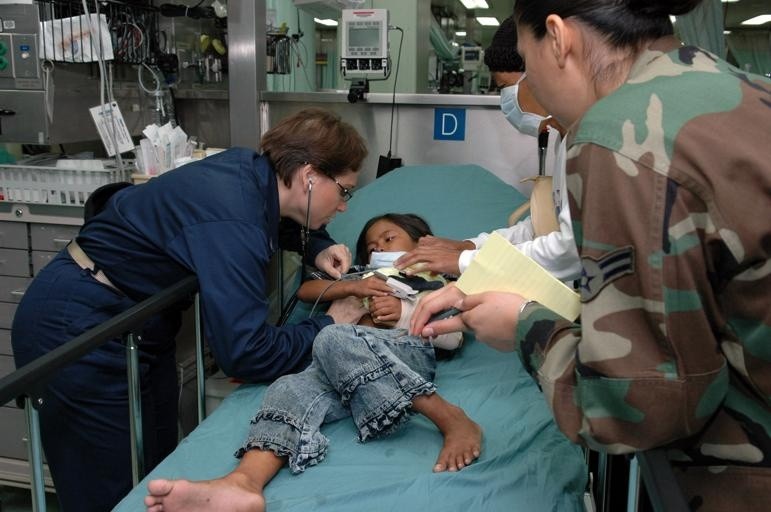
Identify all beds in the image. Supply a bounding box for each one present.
[0,164,692,512]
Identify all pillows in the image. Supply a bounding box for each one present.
[325,164,529,267]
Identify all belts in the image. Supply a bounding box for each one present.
[63,236,115,289]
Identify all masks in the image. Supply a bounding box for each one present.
[500,74,554,137]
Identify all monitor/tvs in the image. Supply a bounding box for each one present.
[462,46,481,71]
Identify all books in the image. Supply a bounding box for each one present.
[462,232,581,323]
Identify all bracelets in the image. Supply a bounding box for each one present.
[520,299,537,314]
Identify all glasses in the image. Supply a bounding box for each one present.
[325,173,353,204]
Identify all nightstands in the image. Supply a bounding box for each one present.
[204,370,241,417]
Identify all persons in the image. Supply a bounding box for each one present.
[10,107,369,512]
[393,16,584,282]
[454,0,771,512]
[144,213,483,512]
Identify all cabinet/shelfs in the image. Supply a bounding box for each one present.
[0,221,209,496]
